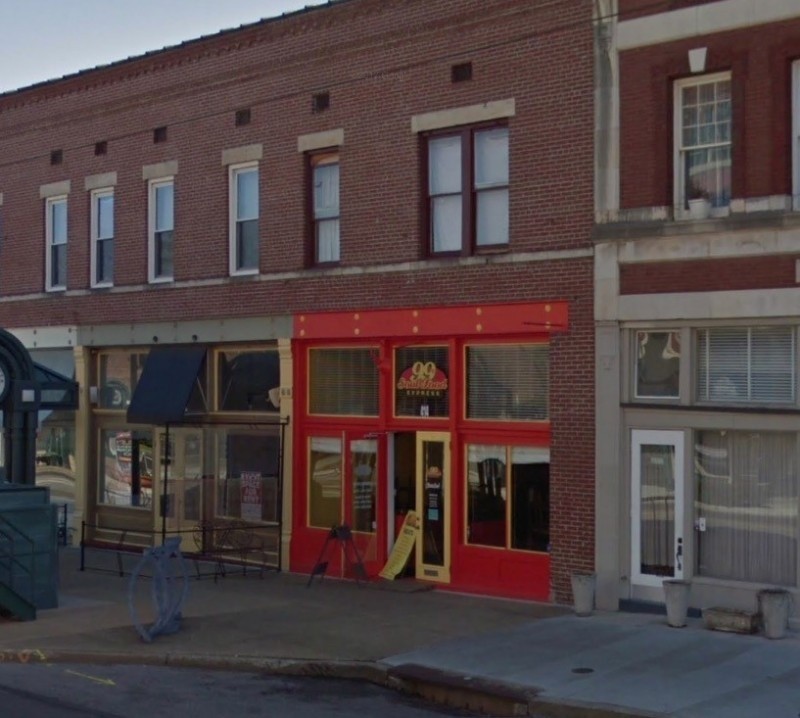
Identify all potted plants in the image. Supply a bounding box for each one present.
[687,186,712,221]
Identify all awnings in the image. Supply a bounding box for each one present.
[126,344,207,426]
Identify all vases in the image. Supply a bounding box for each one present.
[570,574,596,617]
[662,579,692,626]
[758,590,791,639]
[701,605,760,635]
